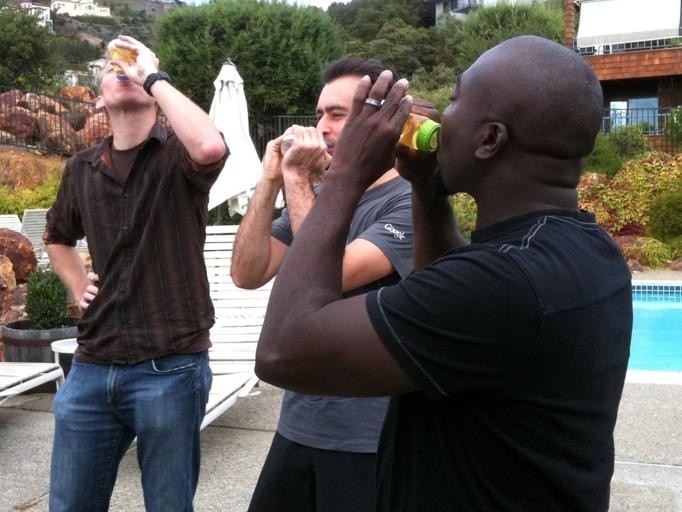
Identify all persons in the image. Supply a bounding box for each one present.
[252,34,634,511]
[230,54,425,512]
[44,34,232,511]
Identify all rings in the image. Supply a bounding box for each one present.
[364,96,382,111]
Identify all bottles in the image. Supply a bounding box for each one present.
[106,38,136,81]
[396,112,441,155]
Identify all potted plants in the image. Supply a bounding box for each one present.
[1,269,84,399]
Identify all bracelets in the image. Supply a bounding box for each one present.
[142,70,174,97]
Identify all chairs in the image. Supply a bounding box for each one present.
[1,209,49,270]
[51,221,277,457]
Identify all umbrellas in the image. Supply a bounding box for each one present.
[204,57,264,219]
